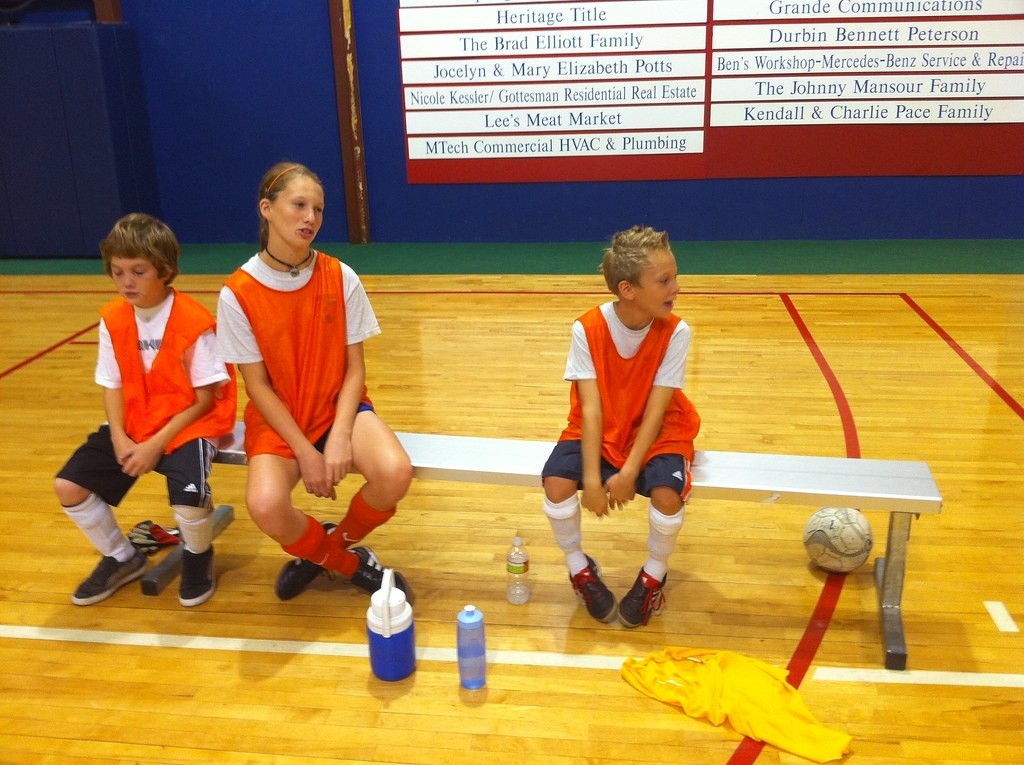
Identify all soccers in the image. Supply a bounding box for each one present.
[805,508,874,572]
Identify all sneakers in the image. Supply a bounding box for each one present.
[345,546,406,598]
[569,554,617,624]
[617,566,667,628]
[275,521,341,600]
[179,544,215,606]
[72,542,146,605]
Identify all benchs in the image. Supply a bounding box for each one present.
[102,422,942,670]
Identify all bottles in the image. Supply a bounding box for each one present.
[455,601,485,691]
[507,535,530,607]
[368,569,416,681]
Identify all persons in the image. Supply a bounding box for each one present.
[542,227,701,627]
[217,160,415,605]
[55,212,238,606]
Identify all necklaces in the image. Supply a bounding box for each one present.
[265,246,311,277]
